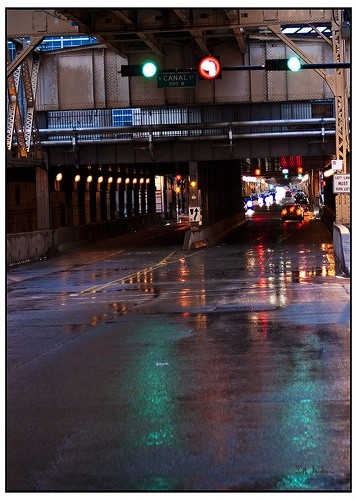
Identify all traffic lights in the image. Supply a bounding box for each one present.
[265,57,301,71]
[197,56,222,80]
[121,62,157,78]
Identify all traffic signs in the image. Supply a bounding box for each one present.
[156,72,197,88]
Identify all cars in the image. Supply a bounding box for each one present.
[294,192,307,203]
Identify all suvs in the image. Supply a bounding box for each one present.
[281,204,304,223]
